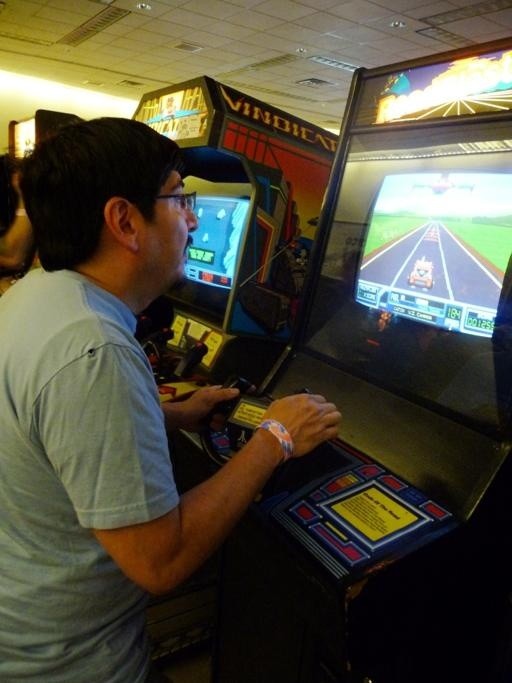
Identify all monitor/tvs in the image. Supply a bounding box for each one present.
[183,193,250,290]
[352,170,512,339]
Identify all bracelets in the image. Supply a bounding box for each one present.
[255,419,295,461]
[15,208,28,217]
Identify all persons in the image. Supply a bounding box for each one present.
[1,116,346,683]
[0,153,36,279]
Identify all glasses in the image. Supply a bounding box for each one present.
[155,191,196,211]
